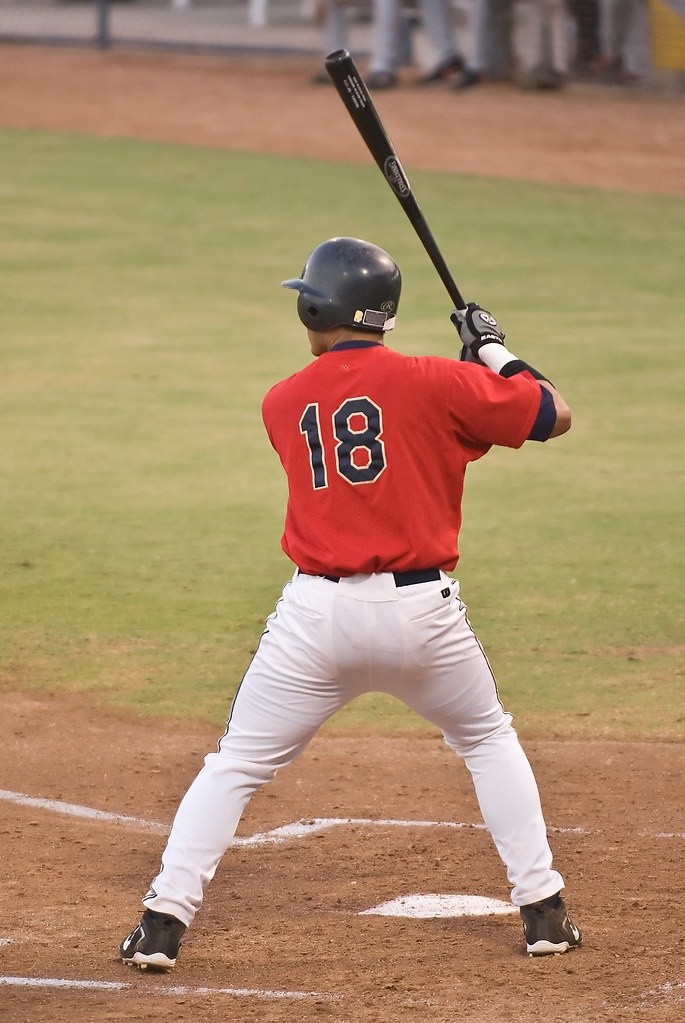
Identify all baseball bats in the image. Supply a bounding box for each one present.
[323,46,467,310]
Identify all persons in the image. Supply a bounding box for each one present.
[119,235,583,975]
[302,0,649,93]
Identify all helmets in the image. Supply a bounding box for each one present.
[281,236,402,332]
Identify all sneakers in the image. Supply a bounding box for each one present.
[120,907,187,968]
[520,898,583,954]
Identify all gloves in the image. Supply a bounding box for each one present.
[449,301,506,369]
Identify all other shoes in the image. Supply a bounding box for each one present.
[364,71,396,89]
[313,71,332,85]
[418,55,482,91]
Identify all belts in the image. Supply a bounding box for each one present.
[298,566,441,587]
